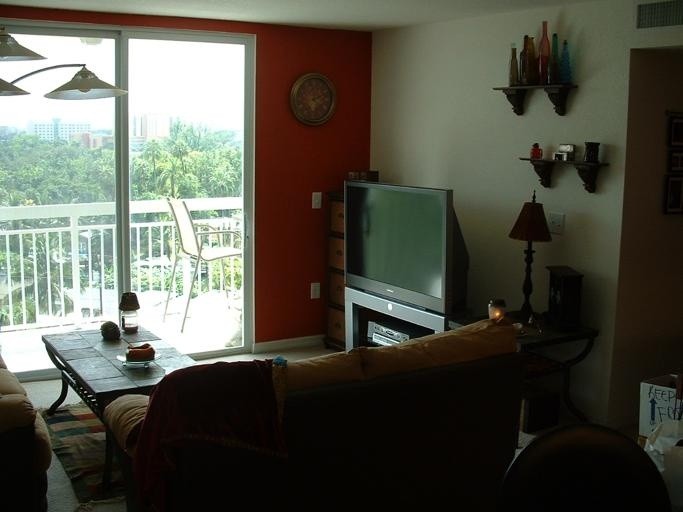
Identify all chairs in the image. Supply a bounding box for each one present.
[499,424,672,511]
[162,196,243,333]
[0,358,51,512]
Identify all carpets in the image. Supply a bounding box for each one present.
[39,400,129,503]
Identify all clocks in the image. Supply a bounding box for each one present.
[290,73,338,126]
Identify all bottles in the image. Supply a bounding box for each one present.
[509,21,571,85]
[530,143,541,159]
[119,292,140,333]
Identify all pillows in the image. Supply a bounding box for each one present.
[358,319,518,379]
[285,351,363,388]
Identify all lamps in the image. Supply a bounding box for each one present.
[488,298,506,324]
[0,24,129,100]
[508,190,553,326]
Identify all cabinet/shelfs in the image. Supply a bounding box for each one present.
[493,84,609,193]
[323,192,344,351]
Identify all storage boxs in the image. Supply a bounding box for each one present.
[637,373,683,511]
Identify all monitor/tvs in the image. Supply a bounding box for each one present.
[343,181,469,317]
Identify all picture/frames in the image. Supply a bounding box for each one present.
[663,116,683,216]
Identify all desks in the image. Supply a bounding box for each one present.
[447,310,599,423]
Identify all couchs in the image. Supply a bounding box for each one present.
[103,319,528,512]
